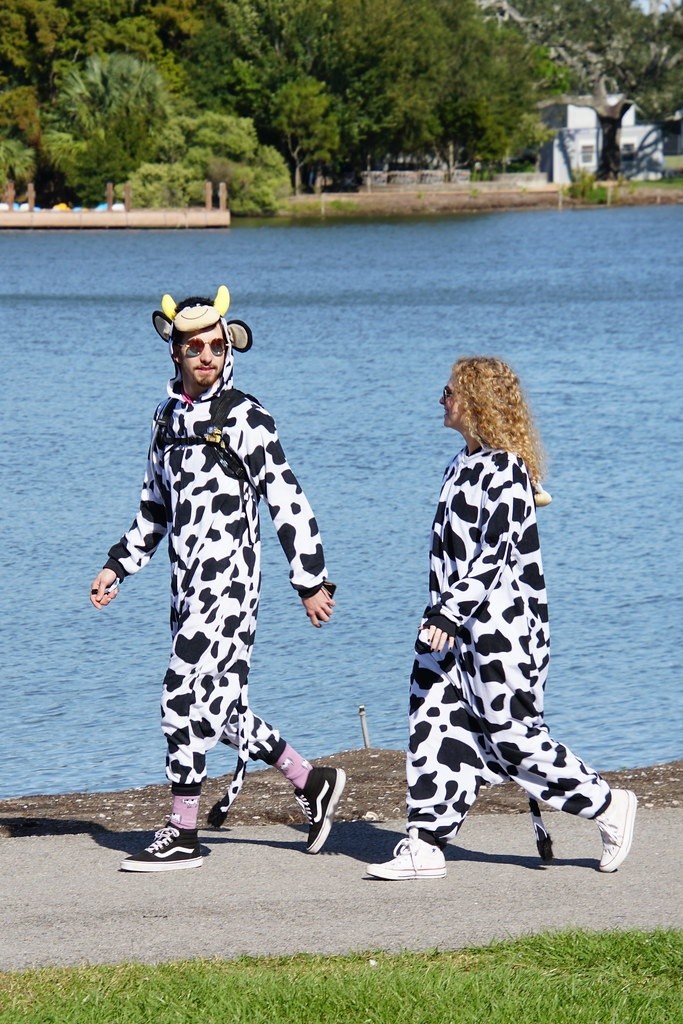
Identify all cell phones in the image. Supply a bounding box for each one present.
[307,581,337,620]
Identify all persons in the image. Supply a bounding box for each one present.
[366,357,639,883]
[87,282,348,872]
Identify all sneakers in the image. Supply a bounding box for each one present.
[119,821,203,871]
[294,767,346,853]
[595,789,637,872]
[365,838,447,879]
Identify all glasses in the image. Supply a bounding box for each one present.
[176,338,227,357]
[443,389,453,402]
